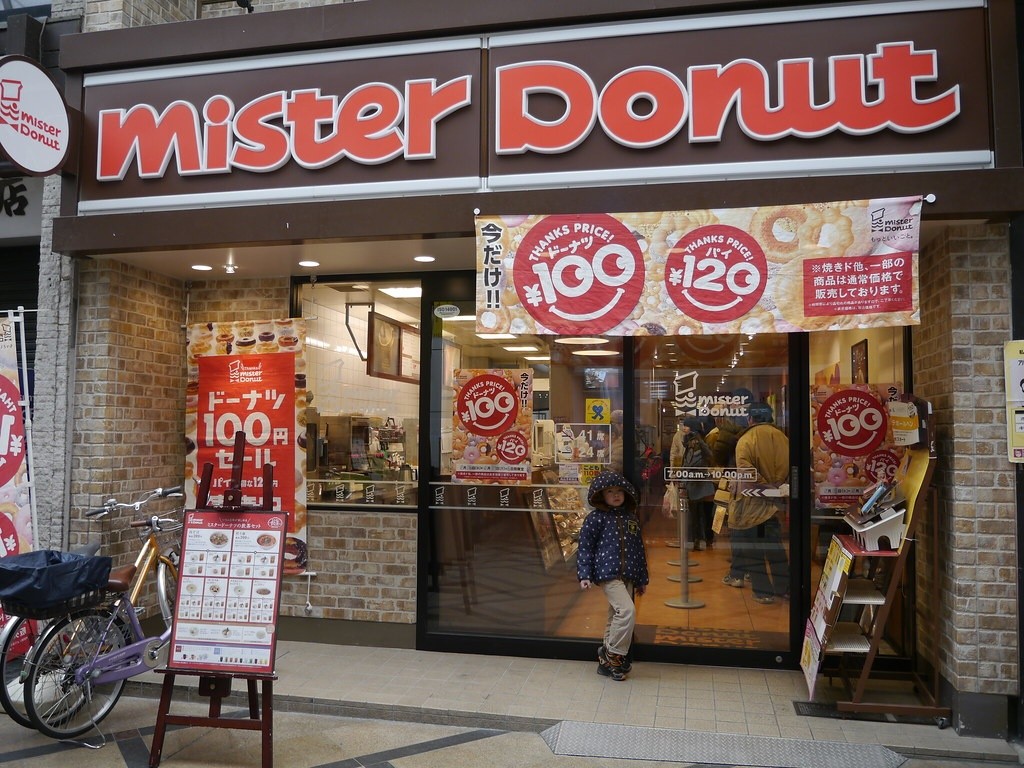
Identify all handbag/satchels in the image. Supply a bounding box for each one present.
[662,482,680,519]
[639,447,663,480]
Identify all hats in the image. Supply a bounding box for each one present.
[610,409,624,424]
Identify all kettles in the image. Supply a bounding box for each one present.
[398,465,417,486]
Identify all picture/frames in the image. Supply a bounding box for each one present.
[850,338,868,384]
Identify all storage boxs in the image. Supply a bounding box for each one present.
[0,550,111,621]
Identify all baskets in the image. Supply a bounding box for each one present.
[0,548,113,621]
[137,507,186,564]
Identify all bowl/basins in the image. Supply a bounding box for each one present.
[255,534,278,551]
[209,532,229,549]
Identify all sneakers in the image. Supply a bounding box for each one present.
[597,646,626,681]
[596,661,632,676]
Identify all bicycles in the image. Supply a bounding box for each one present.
[0,485,188,746]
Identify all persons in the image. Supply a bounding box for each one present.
[577,471,649,681]
[598,410,623,476]
[670,388,789,604]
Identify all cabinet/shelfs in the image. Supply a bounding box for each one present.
[817,393,951,717]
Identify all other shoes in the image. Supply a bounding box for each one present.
[722,574,746,587]
[697,540,708,551]
[688,542,695,552]
[751,592,775,604]
